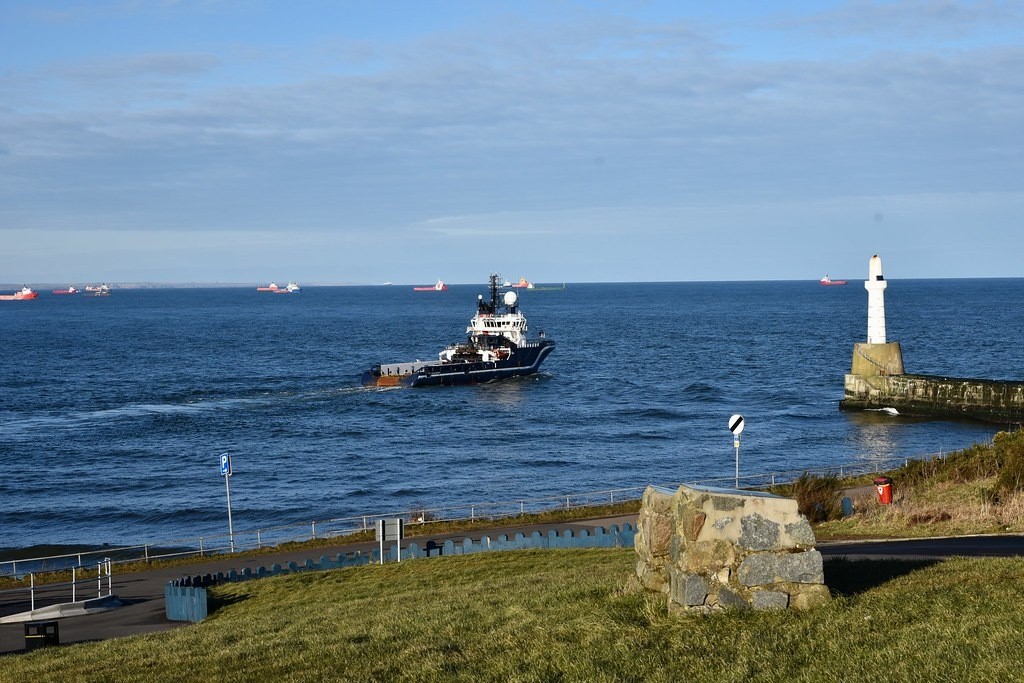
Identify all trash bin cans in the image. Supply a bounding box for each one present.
[873,476,892,504]
[24,619,59,648]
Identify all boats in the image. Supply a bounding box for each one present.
[84,289,111,296]
[820,274,847,285]
[257,282,277,291]
[53,286,81,293]
[503,280,512,287]
[0,285,37,301]
[384,281,392,285]
[527,282,535,288]
[86,283,109,291]
[511,279,528,288]
[414,280,448,290]
[273,281,302,294]
[361,274,557,388]
